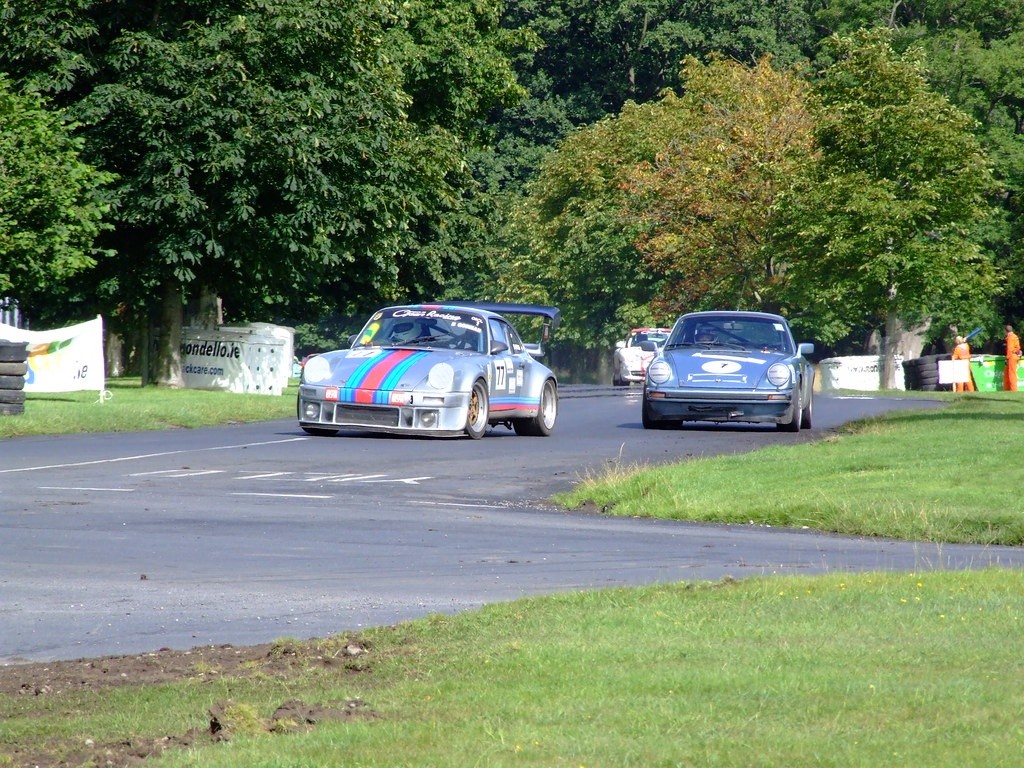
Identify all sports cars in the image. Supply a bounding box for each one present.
[613,328,671,386]
[639,310,816,433]
[297,302,561,440]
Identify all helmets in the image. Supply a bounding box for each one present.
[393,317,422,341]
[693,326,718,343]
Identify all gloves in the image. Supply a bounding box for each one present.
[1005,357,1008,365]
[1015,350,1020,355]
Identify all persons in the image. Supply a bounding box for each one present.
[951,335,975,392]
[1003,324,1020,392]
[392,315,423,344]
[694,324,720,345]
